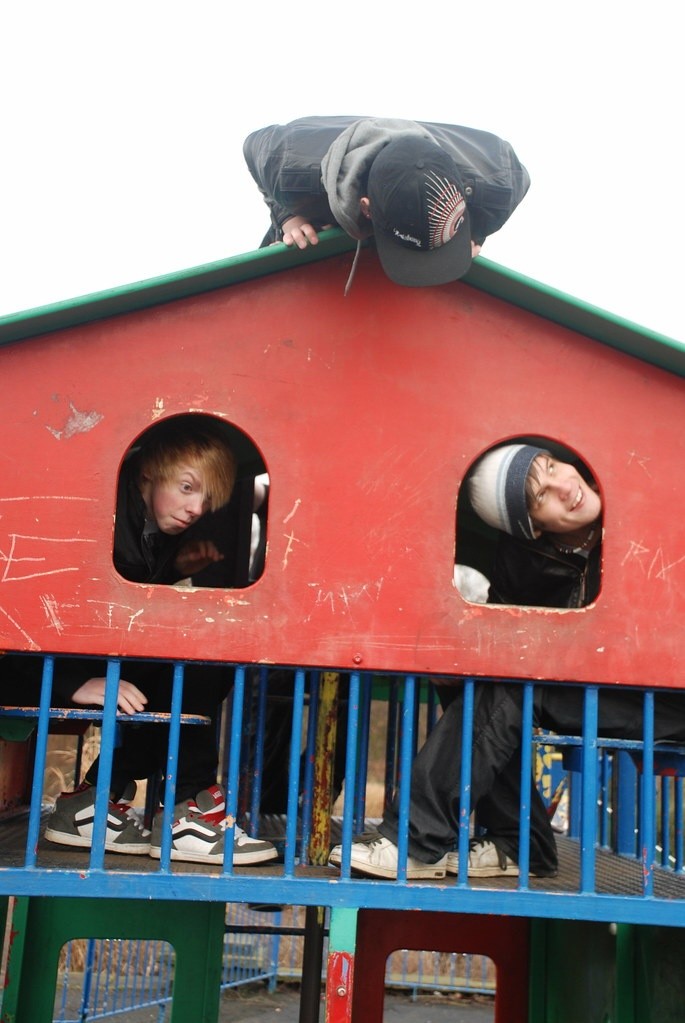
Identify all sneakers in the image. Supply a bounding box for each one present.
[445,835,538,877]
[44,786,152,853]
[149,784,278,866]
[329,837,446,879]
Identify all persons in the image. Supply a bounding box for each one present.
[224,482,348,816]
[0,428,277,866]
[241,116,531,295]
[329,445,683,883]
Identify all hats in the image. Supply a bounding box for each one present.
[368,135,472,287]
[468,444,544,540]
